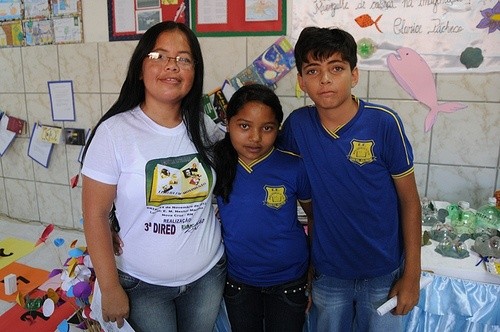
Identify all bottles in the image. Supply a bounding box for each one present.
[452,201,478,236]
[475,197,500,240]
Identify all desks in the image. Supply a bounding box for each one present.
[402,201,500,332]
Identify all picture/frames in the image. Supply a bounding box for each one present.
[191,1,287,37]
[107,0,191,43]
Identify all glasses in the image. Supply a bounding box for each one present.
[145,52,199,69]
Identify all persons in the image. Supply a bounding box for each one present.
[107,80,317,332]
[75,19,231,332]
[273,24,422,332]
